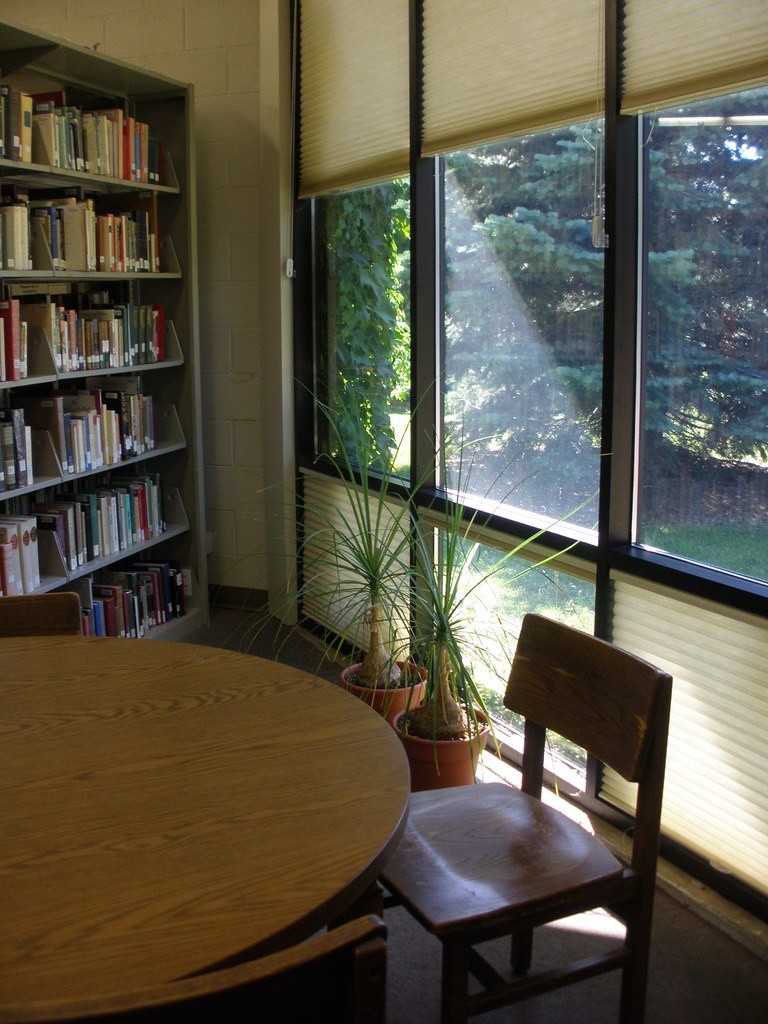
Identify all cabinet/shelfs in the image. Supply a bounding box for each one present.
[0,16,211,644]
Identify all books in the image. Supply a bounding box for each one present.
[0,84,187,638]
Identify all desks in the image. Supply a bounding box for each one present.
[0,630,413,1024]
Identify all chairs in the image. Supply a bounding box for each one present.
[375,612,672,1024]
[0,592,82,638]
[0,912,388,1024]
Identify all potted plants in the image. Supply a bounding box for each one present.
[230,387,556,794]
